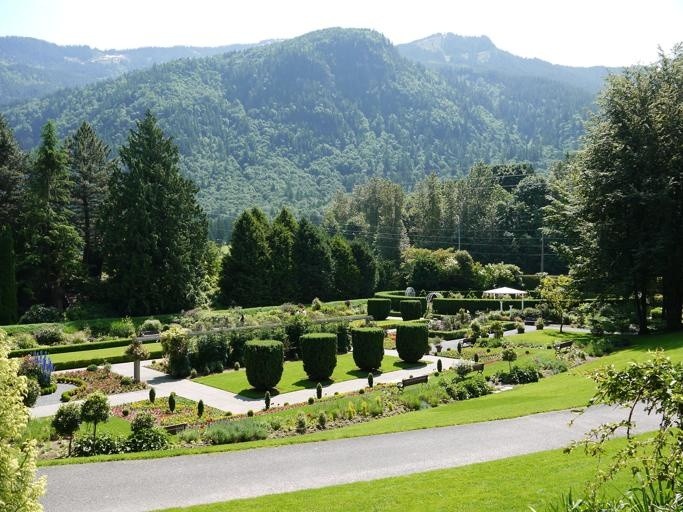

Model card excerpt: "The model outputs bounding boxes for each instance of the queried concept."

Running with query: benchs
[459,364,484,380]
[396,375,428,393]
[163,423,188,436]
[555,340,574,354]
